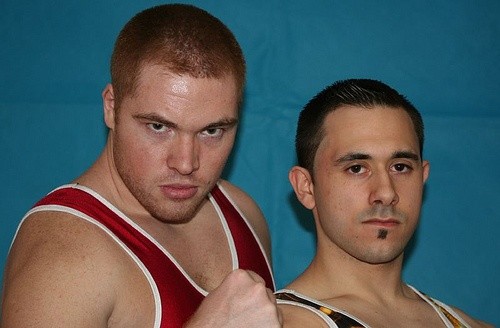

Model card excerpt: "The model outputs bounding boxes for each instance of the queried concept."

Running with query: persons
[1,4,284,328]
[273,79,497,328]
